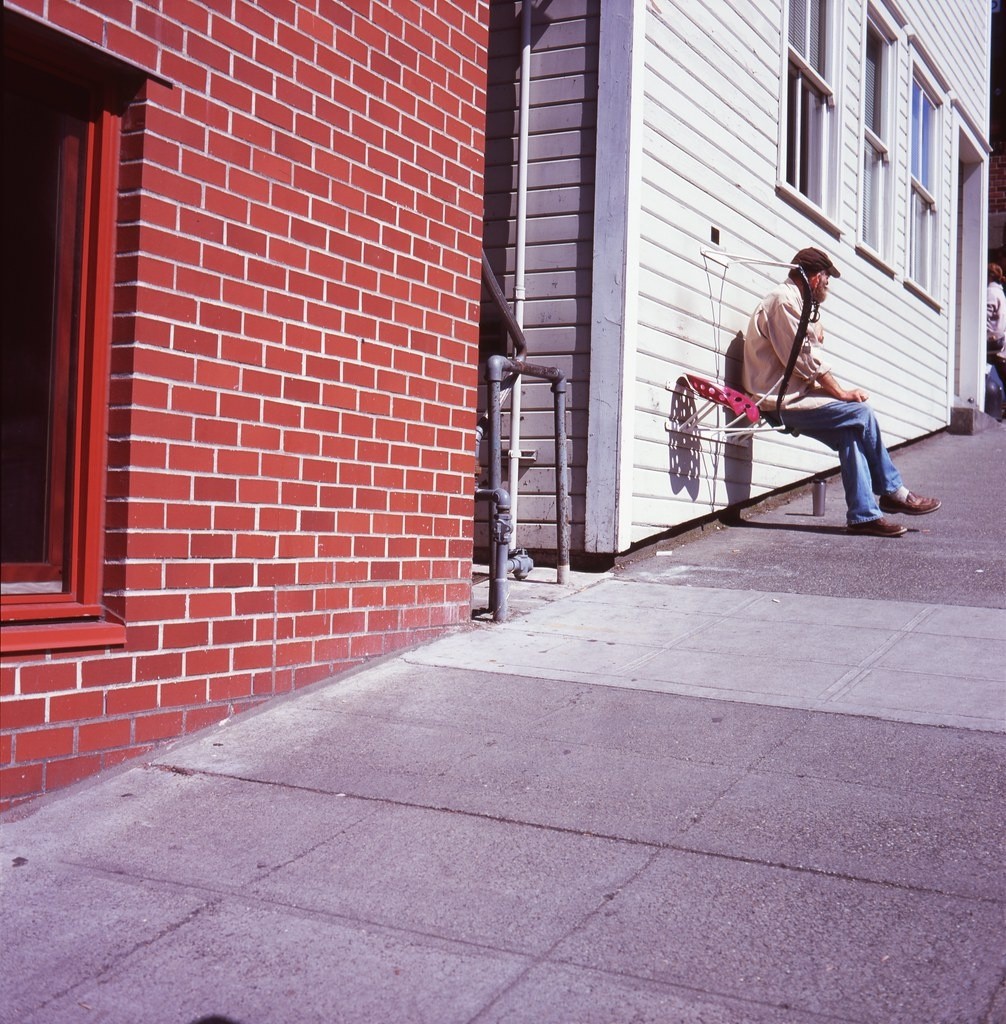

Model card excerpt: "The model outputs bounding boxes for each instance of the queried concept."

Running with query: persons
[987,262,1006,369]
[743,247,943,537]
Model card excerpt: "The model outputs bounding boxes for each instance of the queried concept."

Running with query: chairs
[667,369,793,448]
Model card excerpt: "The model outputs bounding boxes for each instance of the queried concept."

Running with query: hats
[790,247,841,278]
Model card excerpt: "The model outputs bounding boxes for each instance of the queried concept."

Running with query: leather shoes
[878,489,942,515]
[846,517,908,537]
